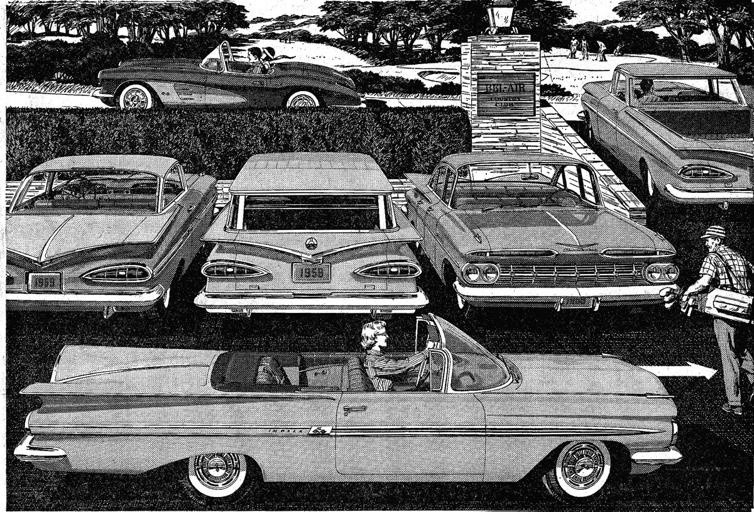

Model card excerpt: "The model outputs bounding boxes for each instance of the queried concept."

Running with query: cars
[577,64,753,211]
[403,152,680,312]
[193,152,430,320]
[6,155,216,321]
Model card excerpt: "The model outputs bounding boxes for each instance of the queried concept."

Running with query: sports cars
[93,40,366,110]
[14,313,683,505]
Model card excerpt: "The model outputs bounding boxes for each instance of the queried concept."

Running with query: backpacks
[690,286,753,324]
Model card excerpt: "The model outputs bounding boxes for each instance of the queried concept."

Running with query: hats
[699,226,727,240]
[261,46,275,60]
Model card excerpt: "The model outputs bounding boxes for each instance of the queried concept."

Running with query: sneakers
[721,390,754,415]
